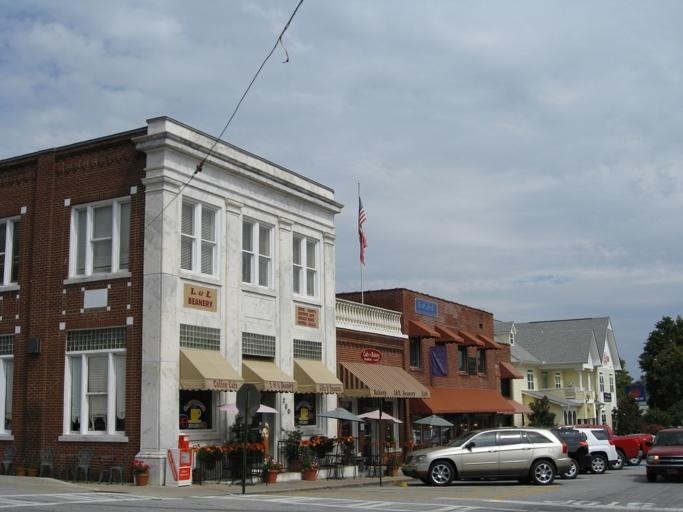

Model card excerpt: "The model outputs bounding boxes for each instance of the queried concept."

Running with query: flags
[357,196,368,267]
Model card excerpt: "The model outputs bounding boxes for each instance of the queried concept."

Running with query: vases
[135,472,149,485]
[16,467,37,477]
[302,470,317,481]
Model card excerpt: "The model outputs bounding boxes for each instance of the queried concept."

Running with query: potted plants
[386,456,400,476]
[263,460,283,484]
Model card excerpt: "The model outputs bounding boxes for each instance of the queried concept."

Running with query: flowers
[280,423,355,470]
[126,459,151,474]
[193,441,266,457]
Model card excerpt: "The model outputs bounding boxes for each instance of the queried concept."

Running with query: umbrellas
[315,406,367,461]
[215,402,280,415]
[412,414,455,427]
[356,409,404,463]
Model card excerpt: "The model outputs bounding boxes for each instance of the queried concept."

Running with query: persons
[258,421,271,459]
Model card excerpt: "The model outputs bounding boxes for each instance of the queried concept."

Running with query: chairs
[38,444,130,485]
[1,445,18,474]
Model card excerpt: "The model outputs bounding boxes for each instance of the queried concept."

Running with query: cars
[401,425,683,486]
